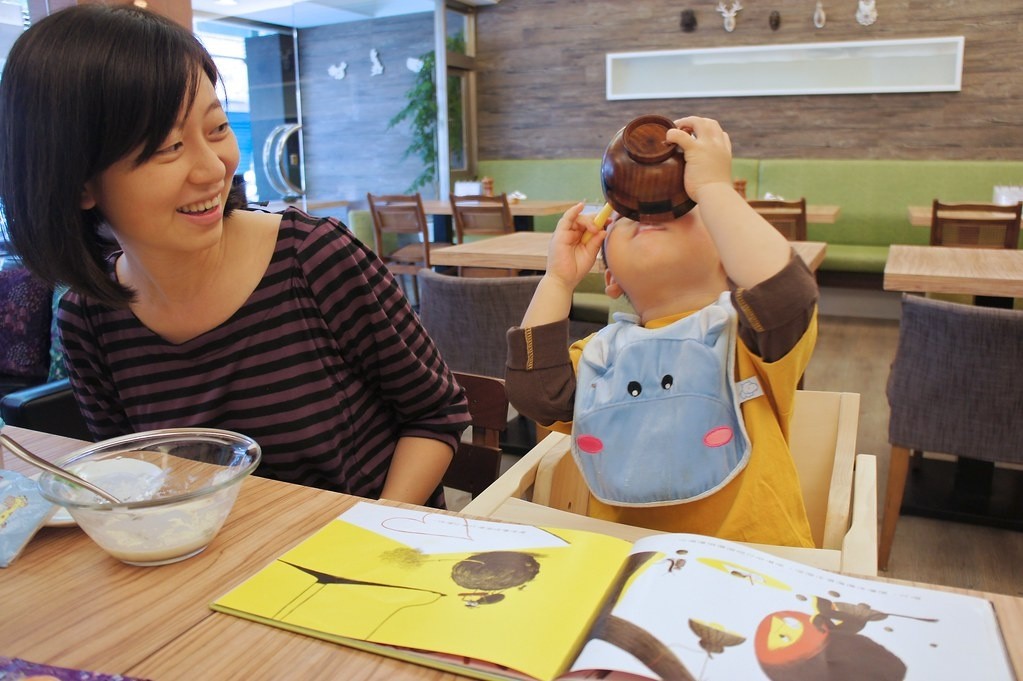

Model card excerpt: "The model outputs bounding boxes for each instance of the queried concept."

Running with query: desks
[429,230,827,453]
[0,424,1023,681]
[752,204,841,225]
[907,205,1023,251]
[388,201,579,242]
[881,243,1023,531]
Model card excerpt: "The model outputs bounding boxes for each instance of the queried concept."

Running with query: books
[212,500,1018,681]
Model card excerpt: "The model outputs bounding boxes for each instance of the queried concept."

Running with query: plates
[27,457,166,527]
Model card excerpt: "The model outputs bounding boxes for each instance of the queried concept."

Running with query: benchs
[453,160,1023,274]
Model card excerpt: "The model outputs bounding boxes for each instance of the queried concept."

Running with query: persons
[503,116,819,549]
[0,4,472,510]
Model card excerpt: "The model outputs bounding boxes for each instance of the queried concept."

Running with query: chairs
[745,198,808,243]
[461,392,878,583]
[877,294,1023,573]
[349,210,419,311]
[418,267,583,450]
[368,190,455,306]
[449,192,524,279]
[929,199,1023,249]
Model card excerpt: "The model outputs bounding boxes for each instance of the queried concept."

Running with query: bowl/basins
[37,428,262,566]
[600,114,697,225]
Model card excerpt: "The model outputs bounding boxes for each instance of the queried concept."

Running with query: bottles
[481,176,494,197]
